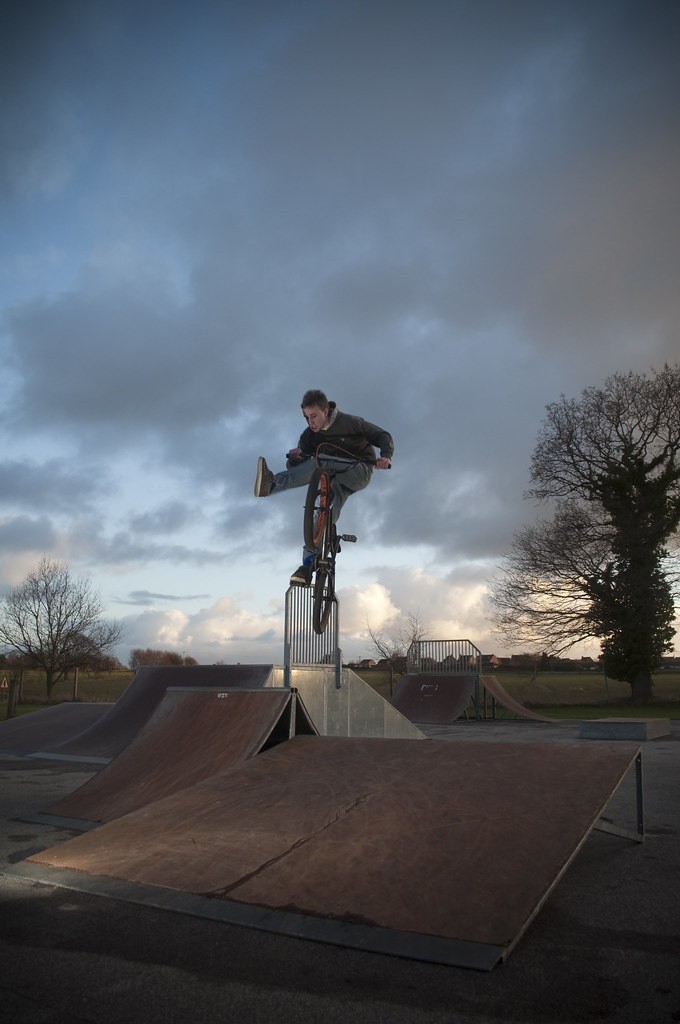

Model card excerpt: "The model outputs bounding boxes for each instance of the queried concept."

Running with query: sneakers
[289,567,309,584]
[254,457,276,497]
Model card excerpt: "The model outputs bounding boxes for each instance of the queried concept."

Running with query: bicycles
[286,441,393,635]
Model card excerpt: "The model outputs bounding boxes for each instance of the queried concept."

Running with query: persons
[253,391,396,584]
